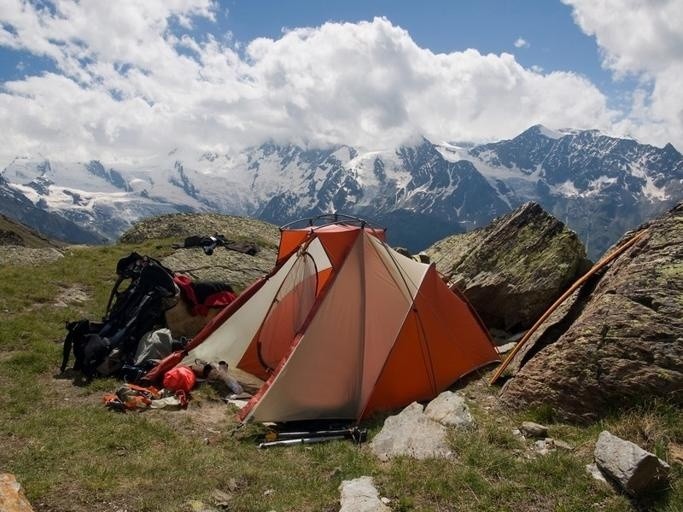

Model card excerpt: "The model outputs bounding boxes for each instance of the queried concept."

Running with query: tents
[140,211,503,430]
